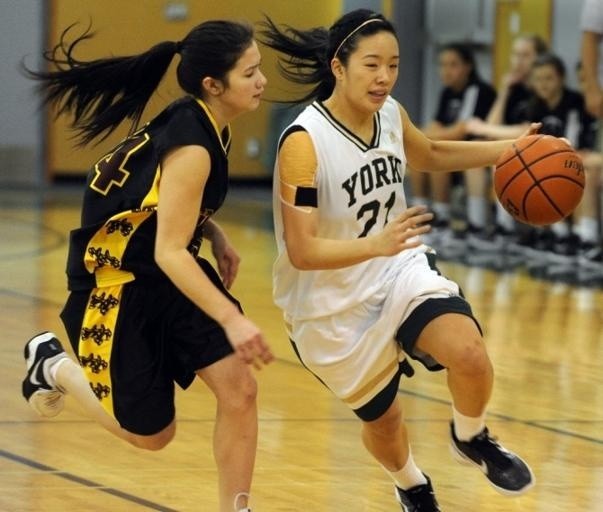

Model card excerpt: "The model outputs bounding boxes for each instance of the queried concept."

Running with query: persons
[271,7,573,512]
[19,17,275,512]
[574,1,603,260]
[509,51,592,254]
[396,38,498,227]
[459,33,549,238]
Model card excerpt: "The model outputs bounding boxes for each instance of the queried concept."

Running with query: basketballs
[495,134,585,225]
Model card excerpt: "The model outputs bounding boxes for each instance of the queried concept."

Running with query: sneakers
[22,330,69,419]
[448,418,536,495]
[419,211,603,270]
[420,232,603,288]
[394,471,441,512]
